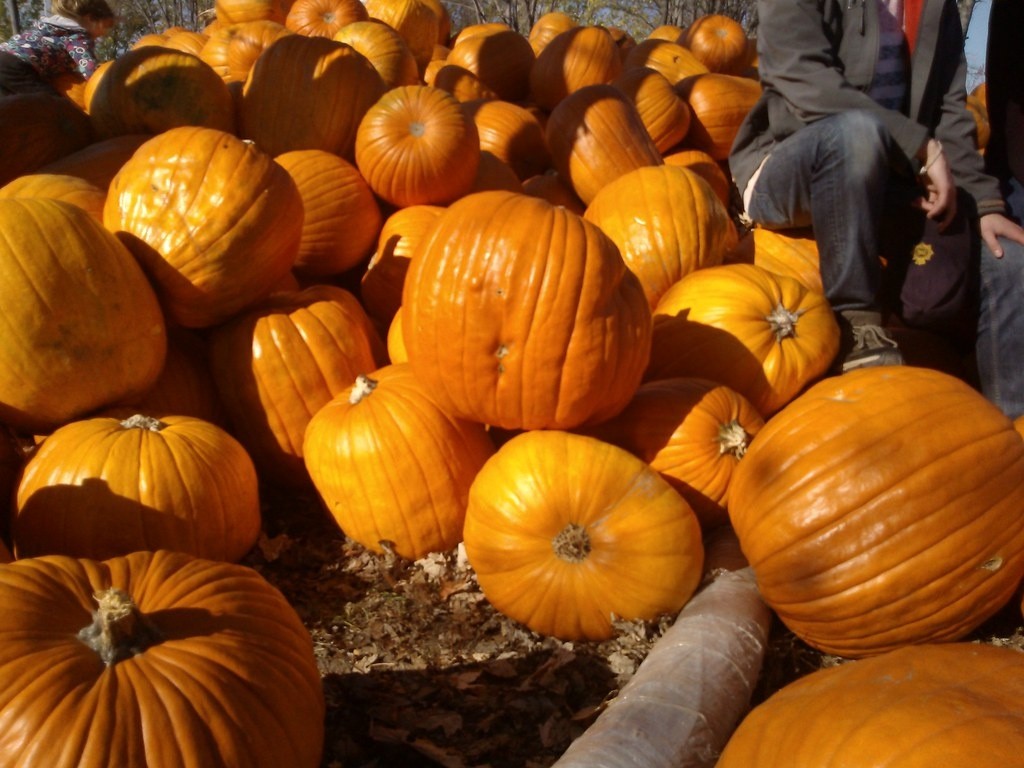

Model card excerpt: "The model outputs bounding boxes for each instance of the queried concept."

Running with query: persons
[0,0,117,98]
[729,0,1024,419]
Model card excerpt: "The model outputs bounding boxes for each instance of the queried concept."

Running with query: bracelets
[918,139,944,176]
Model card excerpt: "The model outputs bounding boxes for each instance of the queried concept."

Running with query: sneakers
[840,310,903,371]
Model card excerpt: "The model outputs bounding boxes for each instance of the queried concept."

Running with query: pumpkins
[0,0,1024,768]
